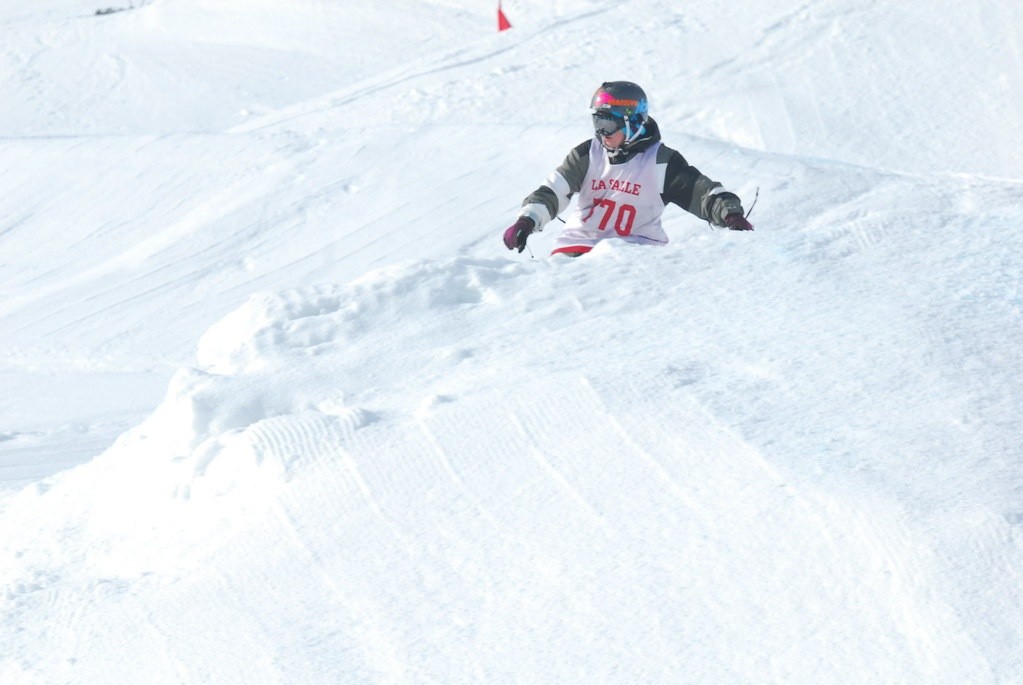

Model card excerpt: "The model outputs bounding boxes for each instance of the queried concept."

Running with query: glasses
[592,113,622,136]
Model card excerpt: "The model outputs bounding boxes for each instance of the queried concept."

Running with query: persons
[503,81,753,255]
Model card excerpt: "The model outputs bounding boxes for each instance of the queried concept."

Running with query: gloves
[503,216,535,253]
[725,213,752,231]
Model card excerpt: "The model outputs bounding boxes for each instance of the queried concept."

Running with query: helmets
[590,81,648,138]
